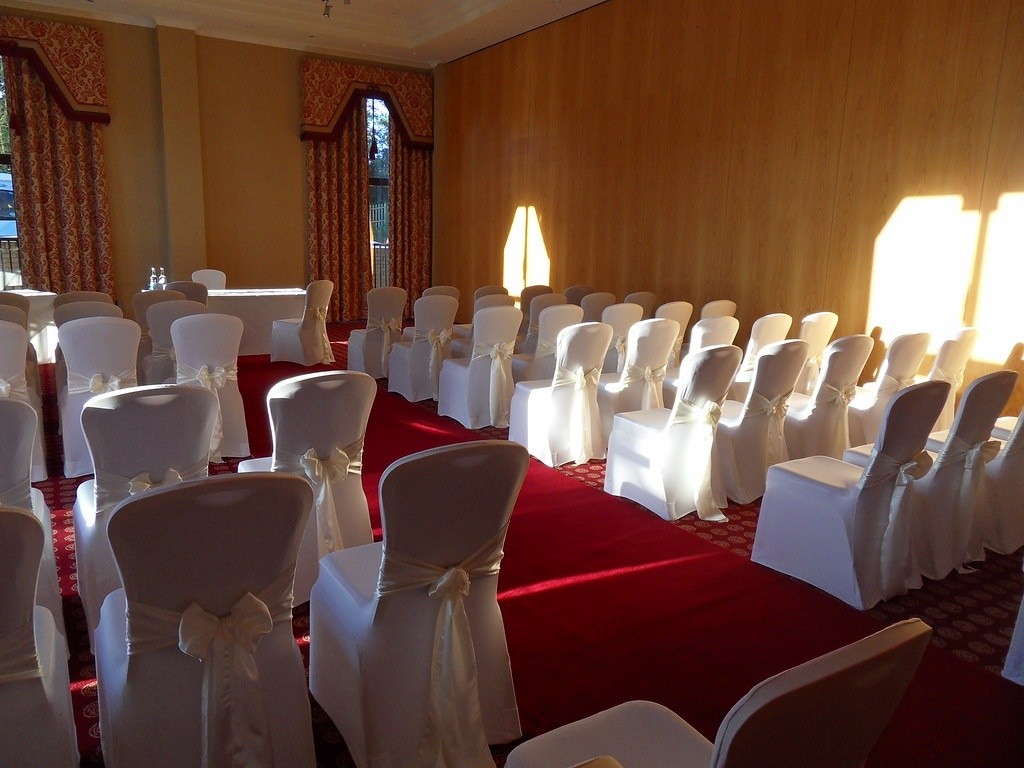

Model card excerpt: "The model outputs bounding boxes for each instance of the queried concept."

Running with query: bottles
[150,268,158,290]
[159,268,168,290]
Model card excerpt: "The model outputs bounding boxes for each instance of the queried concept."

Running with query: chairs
[0,270,1024,611]
[309,440,531,768]
[502,618,934,768]
[1,504,80,768]
[93,471,319,768]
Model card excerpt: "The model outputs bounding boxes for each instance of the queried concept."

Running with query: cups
[154,283,163,291]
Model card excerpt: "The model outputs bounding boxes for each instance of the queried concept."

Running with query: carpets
[213,359,1024,768]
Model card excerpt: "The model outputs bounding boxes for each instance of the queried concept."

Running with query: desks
[1,290,58,339]
[202,288,306,357]
